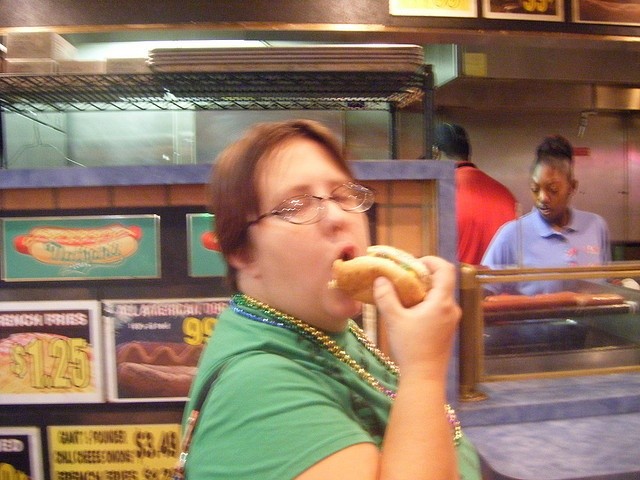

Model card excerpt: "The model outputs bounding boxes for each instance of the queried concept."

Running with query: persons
[479,133,611,340]
[179,117,481,480]
[431,122,517,268]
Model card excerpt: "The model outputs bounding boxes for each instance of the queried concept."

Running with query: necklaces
[229,288,462,448]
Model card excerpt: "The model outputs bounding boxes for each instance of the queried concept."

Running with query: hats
[418,122,469,159]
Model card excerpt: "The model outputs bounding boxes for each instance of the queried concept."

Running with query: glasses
[242,181,376,224]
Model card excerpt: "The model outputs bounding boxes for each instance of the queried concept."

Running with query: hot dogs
[12,224,141,266]
[325,244,431,306]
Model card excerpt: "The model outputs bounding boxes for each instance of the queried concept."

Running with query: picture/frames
[387,0,482,30]
[482,0,568,32]
[570,1,640,36]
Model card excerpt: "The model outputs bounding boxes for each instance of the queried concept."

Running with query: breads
[119,340,205,395]
